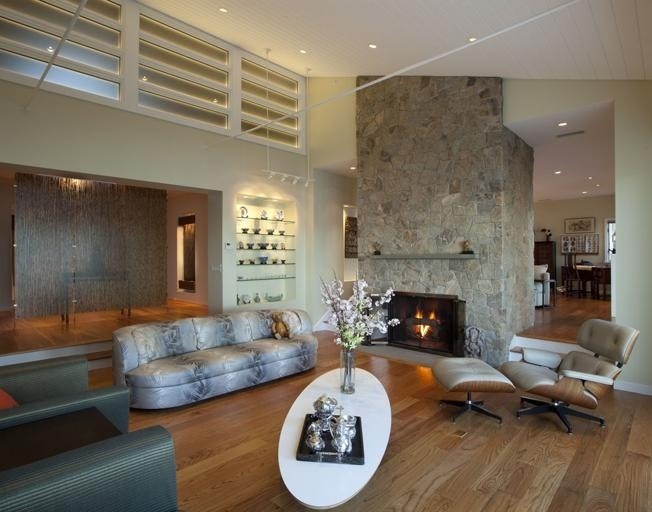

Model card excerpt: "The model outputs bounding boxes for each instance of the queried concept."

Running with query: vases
[339,348,355,394]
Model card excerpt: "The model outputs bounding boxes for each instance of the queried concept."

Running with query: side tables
[0,408,120,472]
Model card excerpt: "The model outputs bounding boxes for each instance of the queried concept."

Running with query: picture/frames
[563,217,596,235]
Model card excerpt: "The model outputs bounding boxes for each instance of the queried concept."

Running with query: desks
[560,265,610,300]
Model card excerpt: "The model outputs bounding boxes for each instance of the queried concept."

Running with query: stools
[431,357,516,424]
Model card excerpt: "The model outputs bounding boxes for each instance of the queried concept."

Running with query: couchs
[111,307,316,410]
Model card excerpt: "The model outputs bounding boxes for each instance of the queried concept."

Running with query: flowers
[314,265,399,391]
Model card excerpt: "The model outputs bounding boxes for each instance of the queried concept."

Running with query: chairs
[499,317,639,434]
[564,253,590,299]
[0,355,128,434]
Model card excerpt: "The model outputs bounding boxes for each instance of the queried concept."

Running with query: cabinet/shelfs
[235,216,296,282]
